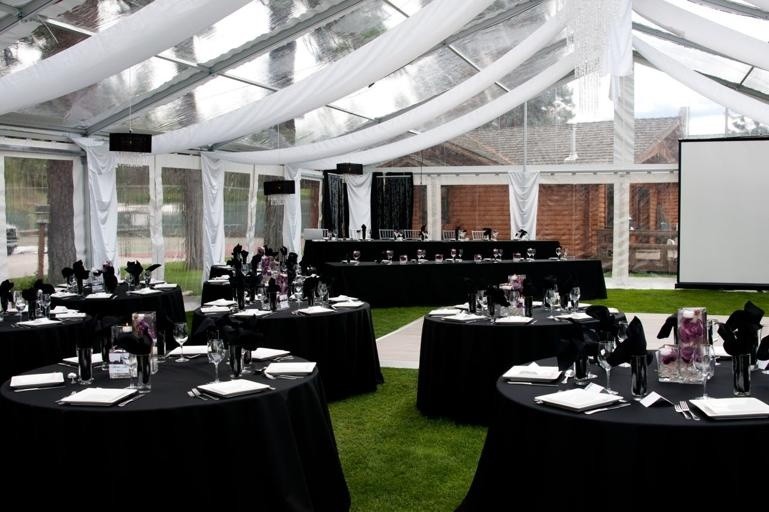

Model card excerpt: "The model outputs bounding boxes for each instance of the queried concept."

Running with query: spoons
[561,368,574,385]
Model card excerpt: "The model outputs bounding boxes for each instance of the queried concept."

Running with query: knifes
[585,403,631,414]
[506,380,559,388]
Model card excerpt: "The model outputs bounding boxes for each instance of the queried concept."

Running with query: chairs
[378,228,489,240]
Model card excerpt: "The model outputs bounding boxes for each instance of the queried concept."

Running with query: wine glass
[9,290,51,320]
[544,287,580,318]
[353,247,568,261]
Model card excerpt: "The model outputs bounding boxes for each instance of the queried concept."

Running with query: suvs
[5,223,18,255]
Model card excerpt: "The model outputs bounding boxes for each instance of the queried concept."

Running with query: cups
[75,321,244,392]
[571,319,753,399]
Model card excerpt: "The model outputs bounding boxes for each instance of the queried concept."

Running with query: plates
[535,389,623,412]
[194,379,271,398]
[8,352,138,405]
[429,300,619,324]
[249,346,319,379]
[689,397,768,419]
[502,364,560,382]
[202,293,364,315]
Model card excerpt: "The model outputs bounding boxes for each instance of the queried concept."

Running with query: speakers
[337,163,363,175]
[263,180,295,195]
[109,132,152,153]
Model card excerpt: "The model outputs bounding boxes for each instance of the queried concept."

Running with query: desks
[304,238,601,304]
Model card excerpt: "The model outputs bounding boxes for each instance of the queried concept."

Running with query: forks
[674,399,699,421]
